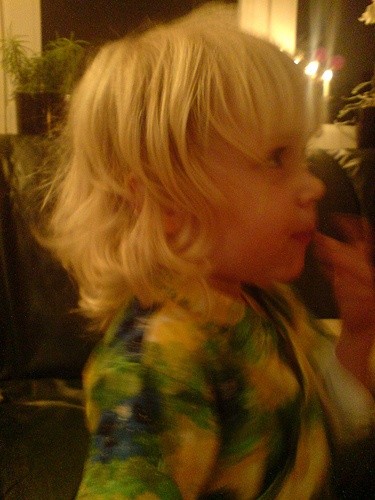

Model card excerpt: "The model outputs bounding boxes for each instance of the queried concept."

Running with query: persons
[26,6,375,500]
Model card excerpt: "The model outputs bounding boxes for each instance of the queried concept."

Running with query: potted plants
[0,22,92,136]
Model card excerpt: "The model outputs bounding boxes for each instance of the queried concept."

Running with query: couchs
[0,132,375,500]
